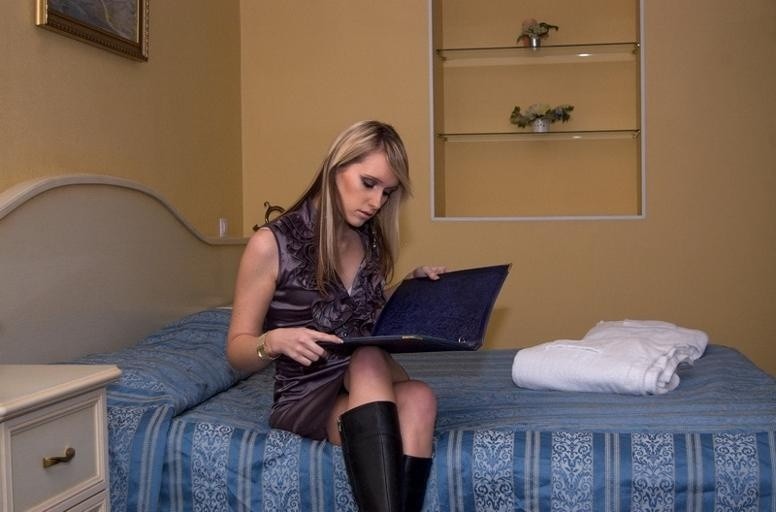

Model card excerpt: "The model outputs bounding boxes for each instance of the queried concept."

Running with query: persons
[225,119,453,512]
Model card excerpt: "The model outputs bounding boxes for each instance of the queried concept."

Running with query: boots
[338,400,434,512]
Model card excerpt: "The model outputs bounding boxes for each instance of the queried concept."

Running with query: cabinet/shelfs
[428,1,645,224]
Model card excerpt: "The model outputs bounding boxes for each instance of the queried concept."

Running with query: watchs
[256,329,281,360]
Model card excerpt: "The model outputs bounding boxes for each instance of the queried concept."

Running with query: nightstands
[1,364,124,512]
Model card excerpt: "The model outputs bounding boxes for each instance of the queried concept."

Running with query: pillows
[68,308,273,414]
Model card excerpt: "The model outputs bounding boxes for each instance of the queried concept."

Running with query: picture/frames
[34,1,149,62]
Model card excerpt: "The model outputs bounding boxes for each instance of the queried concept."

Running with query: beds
[1,176,776,512]
